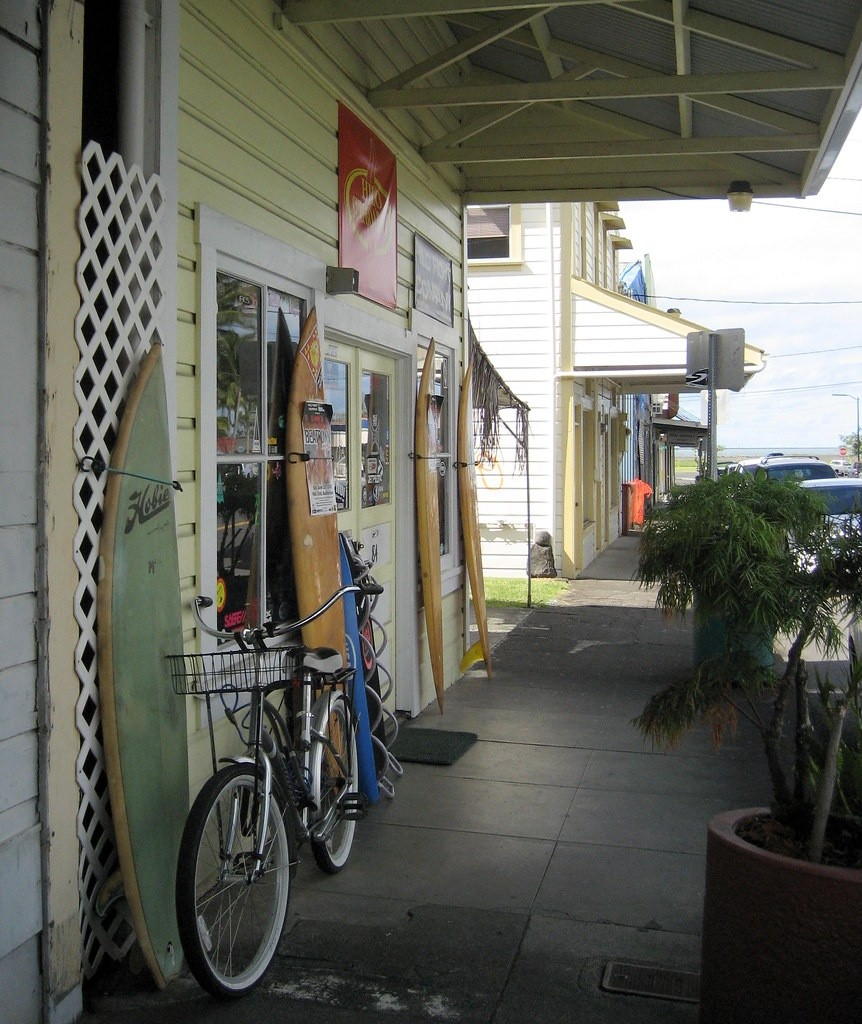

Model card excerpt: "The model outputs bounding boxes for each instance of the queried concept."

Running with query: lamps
[727,180,753,211]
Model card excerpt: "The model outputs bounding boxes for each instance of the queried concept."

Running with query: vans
[831,459,853,474]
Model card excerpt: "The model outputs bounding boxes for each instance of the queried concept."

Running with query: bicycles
[164,580,385,997]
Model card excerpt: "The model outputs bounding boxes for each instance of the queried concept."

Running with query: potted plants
[626,463,862,1024]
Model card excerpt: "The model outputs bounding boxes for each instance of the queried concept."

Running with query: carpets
[387,725,478,768]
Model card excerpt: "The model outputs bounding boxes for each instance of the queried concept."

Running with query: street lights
[832,394,860,461]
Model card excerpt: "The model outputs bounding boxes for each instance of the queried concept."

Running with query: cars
[781,478,862,581]
[695,453,840,481]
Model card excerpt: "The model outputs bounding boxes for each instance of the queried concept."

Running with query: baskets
[164,646,301,695]
